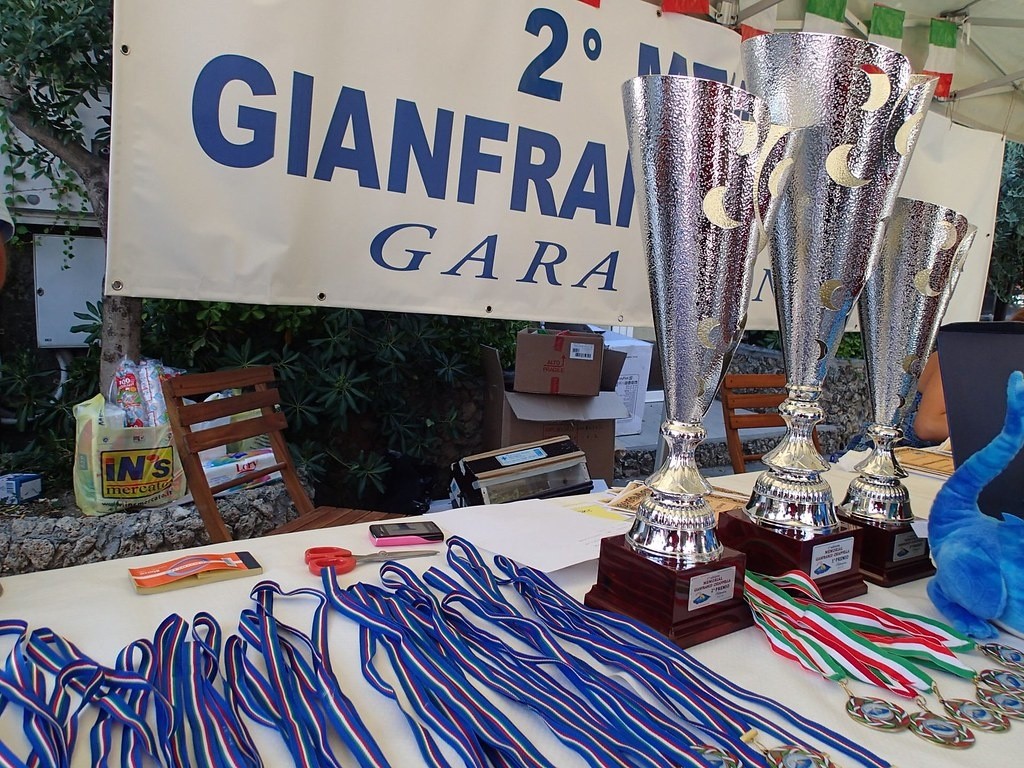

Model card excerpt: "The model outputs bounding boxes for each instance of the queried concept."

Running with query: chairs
[161,366,407,543]
[720,373,821,474]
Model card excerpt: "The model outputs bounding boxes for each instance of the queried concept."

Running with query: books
[128,551,263,597]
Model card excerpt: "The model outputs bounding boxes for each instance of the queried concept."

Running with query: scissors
[305,545,439,576]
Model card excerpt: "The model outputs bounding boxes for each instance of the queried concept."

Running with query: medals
[693,641,1024,768]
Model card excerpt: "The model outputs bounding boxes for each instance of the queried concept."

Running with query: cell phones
[369,521,445,544]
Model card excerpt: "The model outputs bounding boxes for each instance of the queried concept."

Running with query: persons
[828,306,1024,464]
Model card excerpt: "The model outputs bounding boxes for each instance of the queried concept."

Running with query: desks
[0,446,1024,768]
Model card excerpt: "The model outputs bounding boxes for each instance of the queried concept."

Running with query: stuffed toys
[924,367,1024,642]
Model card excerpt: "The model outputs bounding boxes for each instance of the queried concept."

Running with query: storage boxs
[514,327,605,396]
[542,325,654,436]
[459,434,591,504]
[0,473,43,505]
[479,344,632,488]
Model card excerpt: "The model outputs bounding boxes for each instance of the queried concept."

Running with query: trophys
[838,195,980,591]
[584,76,809,649]
[714,29,940,600]
